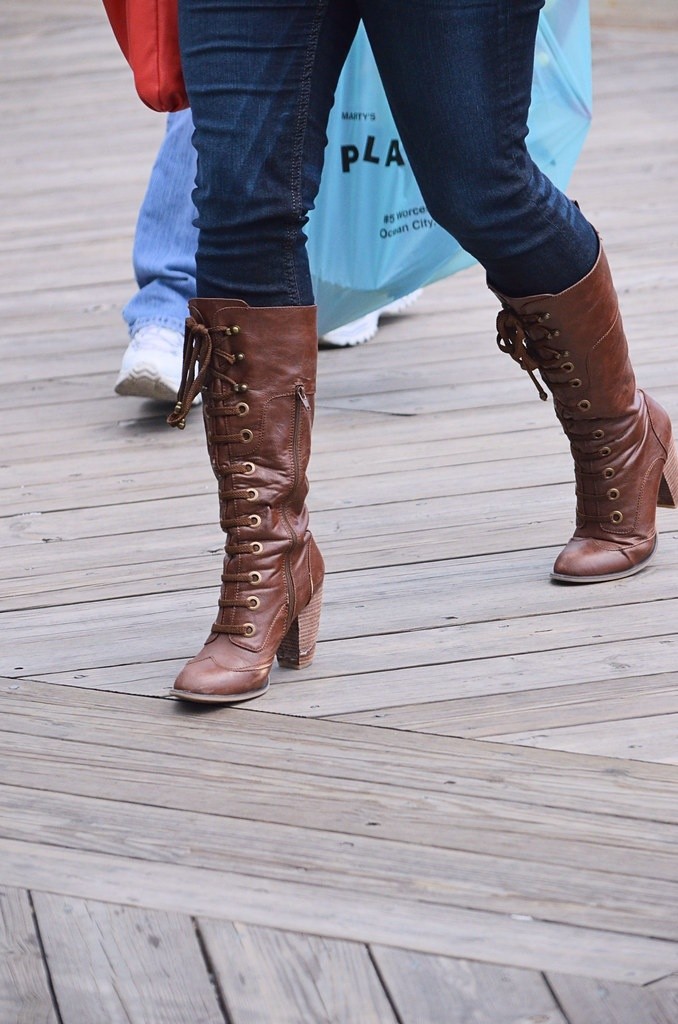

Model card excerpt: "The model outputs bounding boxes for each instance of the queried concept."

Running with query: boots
[487,224,678,584]
[167,300,324,704]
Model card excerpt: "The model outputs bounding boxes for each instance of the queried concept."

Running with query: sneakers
[319,285,423,344]
[115,329,201,407]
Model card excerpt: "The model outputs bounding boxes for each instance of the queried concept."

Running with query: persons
[102,0,677,703]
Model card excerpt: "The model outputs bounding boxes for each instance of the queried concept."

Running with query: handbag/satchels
[101,0,191,113]
